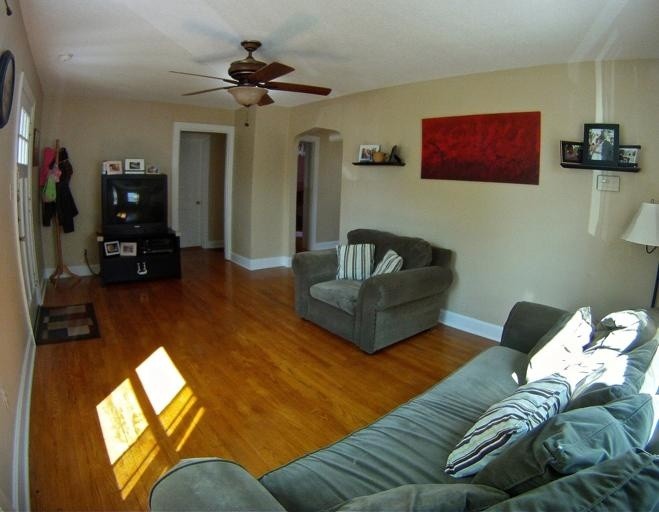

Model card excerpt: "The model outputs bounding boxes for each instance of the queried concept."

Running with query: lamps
[227,41,268,108]
[619,202,659,307]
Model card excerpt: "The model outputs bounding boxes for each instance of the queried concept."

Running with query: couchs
[149,302,659,512]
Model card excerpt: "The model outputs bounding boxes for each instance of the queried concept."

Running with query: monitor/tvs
[101,174,168,236]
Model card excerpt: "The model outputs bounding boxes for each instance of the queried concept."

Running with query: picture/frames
[33,128,41,166]
[582,124,619,167]
[120,242,137,256]
[106,161,122,174]
[561,140,583,165]
[359,145,379,161]
[104,240,120,256]
[618,144,641,167]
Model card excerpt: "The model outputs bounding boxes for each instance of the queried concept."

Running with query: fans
[169,40,331,106]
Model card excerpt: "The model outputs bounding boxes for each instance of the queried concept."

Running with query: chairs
[292,229,453,354]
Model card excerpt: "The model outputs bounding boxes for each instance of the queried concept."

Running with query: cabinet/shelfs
[95,229,181,288]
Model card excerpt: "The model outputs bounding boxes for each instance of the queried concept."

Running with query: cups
[372,151,389,163]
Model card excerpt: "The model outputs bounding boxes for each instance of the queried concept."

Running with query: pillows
[372,249,403,277]
[335,243,376,281]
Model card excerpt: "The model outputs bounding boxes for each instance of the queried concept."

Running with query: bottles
[101,162,107,174]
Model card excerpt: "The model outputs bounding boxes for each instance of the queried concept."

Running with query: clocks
[0,50,16,128]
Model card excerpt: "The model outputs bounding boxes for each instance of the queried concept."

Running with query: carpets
[36,302,101,345]
